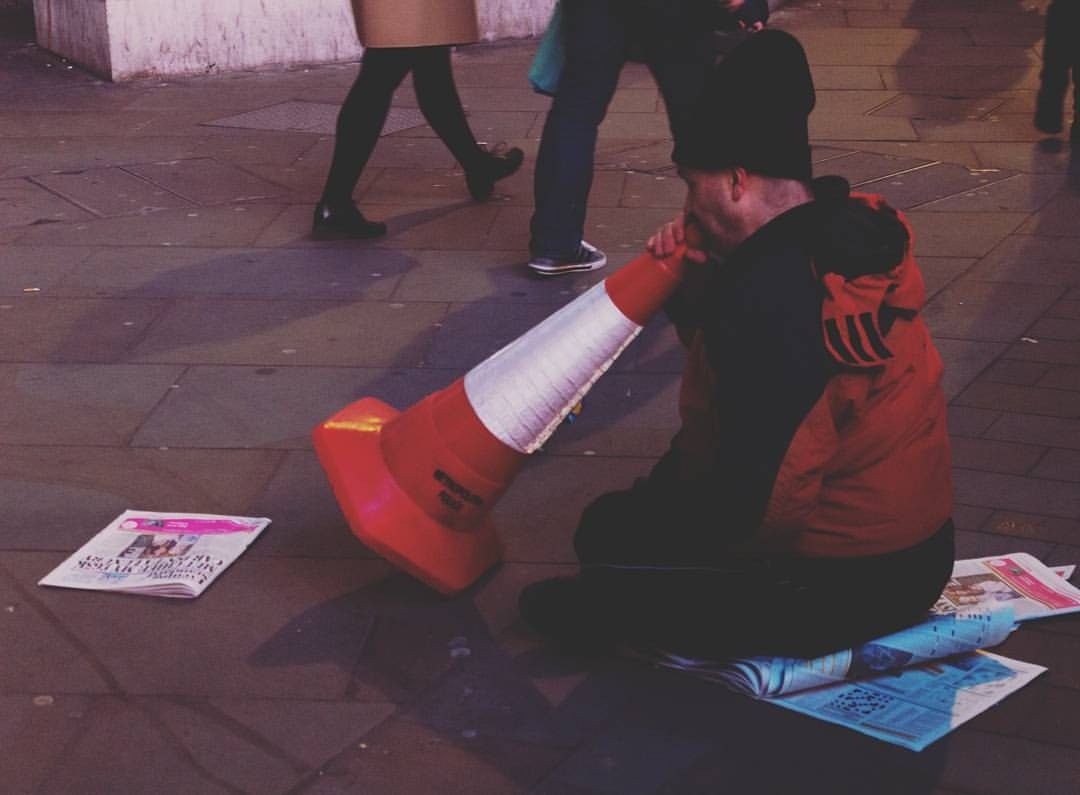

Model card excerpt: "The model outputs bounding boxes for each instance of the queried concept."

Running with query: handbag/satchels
[528,3,565,97]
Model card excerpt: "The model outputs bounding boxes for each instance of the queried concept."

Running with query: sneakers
[312,199,387,240]
[467,141,524,200]
[527,240,608,276]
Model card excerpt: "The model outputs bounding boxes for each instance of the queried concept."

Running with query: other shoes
[1033,95,1066,134]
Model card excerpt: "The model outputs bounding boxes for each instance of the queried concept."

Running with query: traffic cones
[305,218,707,595]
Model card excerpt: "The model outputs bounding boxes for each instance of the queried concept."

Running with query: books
[38,511,272,598]
[657,605,1048,753]
[928,552,1080,621]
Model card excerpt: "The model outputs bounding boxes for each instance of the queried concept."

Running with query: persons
[314,0,523,240]
[944,575,1020,603]
[1035,0,1080,133]
[516,29,955,658]
[529,1,770,274]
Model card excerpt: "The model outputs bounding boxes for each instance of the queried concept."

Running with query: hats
[672,23,817,181]
